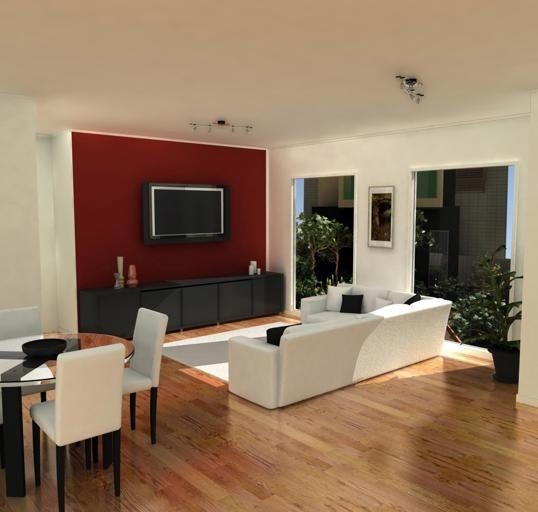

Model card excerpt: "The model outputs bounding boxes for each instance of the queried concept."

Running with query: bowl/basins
[22,352,65,368]
[23,337,67,360]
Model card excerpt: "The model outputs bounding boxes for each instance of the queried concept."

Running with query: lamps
[395,75,424,104]
[186,122,253,135]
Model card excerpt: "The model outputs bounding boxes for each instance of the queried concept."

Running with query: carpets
[162,322,299,384]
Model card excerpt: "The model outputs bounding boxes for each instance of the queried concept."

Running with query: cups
[250,261,257,273]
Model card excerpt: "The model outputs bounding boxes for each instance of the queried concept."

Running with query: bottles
[256,268,260,275]
[249,264,255,275]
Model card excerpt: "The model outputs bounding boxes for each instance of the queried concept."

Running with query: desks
[0,332,135,496]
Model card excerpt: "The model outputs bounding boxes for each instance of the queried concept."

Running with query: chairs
[0,305,46,470]
[29,343,125,512]
[92,307,170,464]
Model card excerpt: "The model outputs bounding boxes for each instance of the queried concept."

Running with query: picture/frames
[367,185,395,249]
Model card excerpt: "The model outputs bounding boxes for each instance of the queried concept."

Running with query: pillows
[325,283,352,311]
[404,294,422,304]
[266,321,302,346]
[375,297,392,309]
[340,294,363,313]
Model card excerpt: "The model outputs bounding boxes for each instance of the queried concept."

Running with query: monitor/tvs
[149,183,224,240]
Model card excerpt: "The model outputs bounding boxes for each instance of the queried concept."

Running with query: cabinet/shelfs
[78,271,283,341]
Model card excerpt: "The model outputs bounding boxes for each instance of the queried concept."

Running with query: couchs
[229,292,453,409]
[301,282,390,323]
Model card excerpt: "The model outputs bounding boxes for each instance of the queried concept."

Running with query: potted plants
[450,243,525,382]
